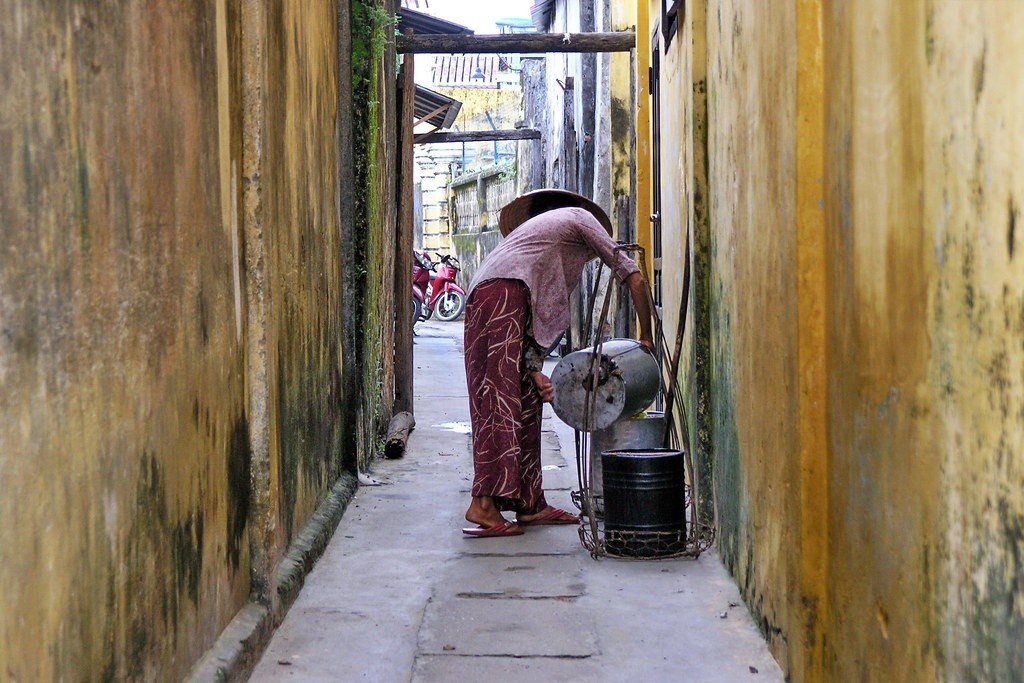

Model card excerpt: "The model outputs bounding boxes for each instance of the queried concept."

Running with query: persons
[464,189,653,536]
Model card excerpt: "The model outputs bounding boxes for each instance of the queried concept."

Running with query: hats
[499,189,613,262]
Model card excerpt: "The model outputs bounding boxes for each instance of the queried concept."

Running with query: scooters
[420,251,467,322]
[410,247,440,337]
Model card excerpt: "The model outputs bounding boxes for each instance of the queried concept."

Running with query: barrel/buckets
[548,337,689,558]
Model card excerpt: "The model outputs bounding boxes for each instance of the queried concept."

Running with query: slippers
[511,508,580,525]
[462,521,525,535]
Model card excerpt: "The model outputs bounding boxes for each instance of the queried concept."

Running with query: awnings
[400,9,475,35]
[414,84,463,143]
